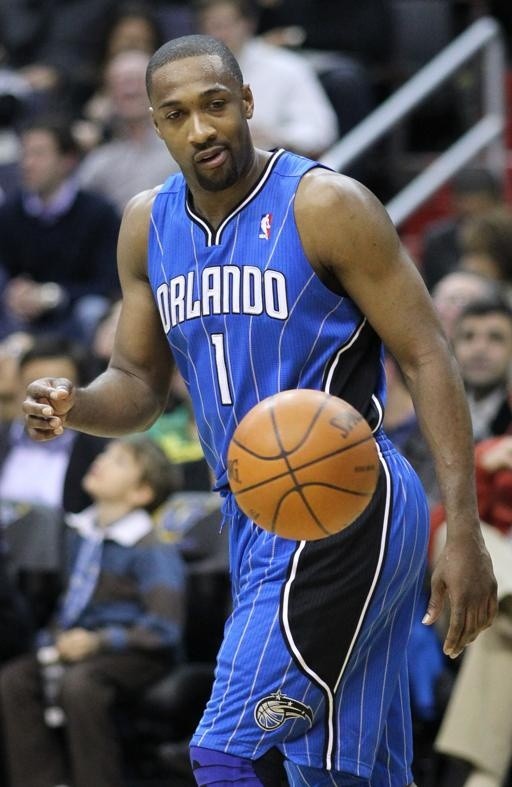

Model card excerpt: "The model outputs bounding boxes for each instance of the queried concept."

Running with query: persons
[0,0,512,787]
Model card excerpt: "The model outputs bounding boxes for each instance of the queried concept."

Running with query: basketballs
[226,388,380,540]
